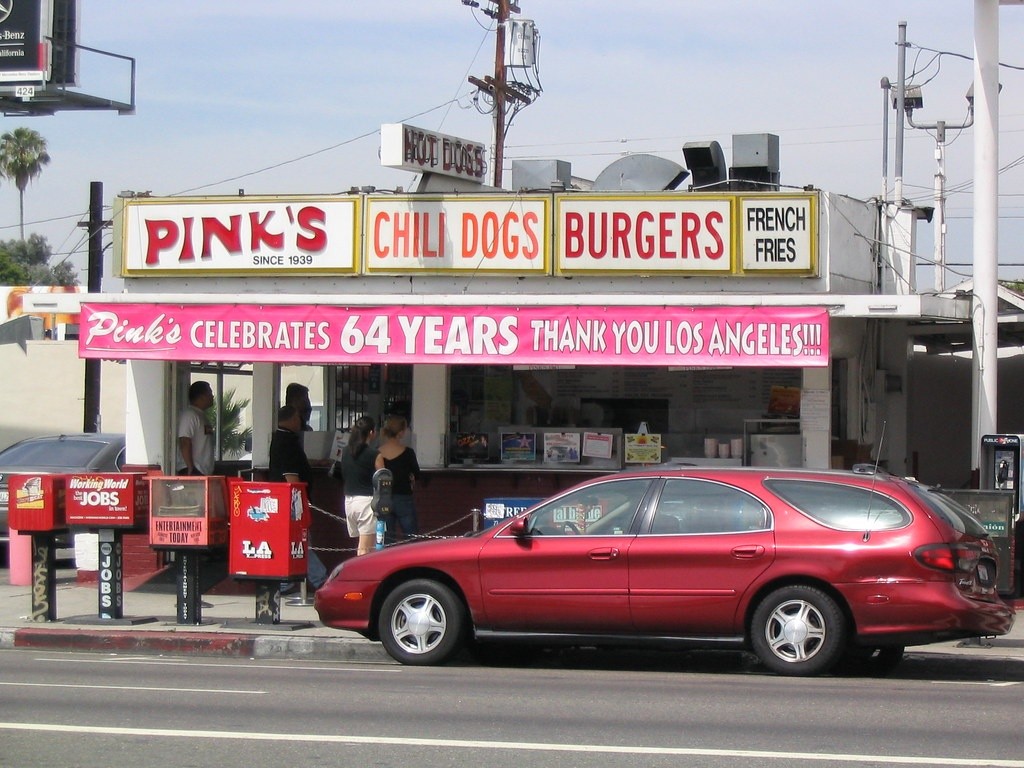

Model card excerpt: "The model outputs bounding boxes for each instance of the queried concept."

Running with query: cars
[315,467,1014,676]
[0,432,129,570]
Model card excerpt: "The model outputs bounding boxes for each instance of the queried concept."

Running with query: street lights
[888,81,1004,293]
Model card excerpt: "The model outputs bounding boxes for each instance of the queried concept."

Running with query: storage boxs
[832,456,844,469]
[769,384,800,417]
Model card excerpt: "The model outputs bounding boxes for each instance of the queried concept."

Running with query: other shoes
[201,601,214,608]
[282,586,298,595]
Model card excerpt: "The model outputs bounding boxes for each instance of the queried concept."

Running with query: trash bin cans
[943,490,1015,594]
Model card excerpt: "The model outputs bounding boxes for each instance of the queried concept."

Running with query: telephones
[994,447,1016,490]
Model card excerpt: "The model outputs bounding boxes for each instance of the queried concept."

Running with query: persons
[284,383,313,430]
[376,413,424,548]
[269,405,327,592]
[177,381,217,562]
[340,415,386,557]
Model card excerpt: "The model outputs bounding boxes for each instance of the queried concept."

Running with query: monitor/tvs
[453,432,489,465]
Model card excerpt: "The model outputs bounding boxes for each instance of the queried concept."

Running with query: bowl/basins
[704,438,743,458]
